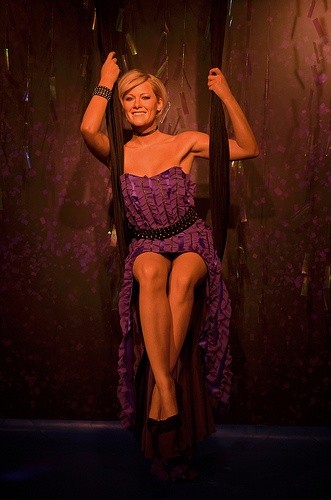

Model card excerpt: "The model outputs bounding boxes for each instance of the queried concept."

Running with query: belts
[133,208,200,240]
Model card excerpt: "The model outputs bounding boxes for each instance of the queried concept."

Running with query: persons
[80,51,260,435]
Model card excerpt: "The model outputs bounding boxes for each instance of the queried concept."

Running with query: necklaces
[131,125,158,136]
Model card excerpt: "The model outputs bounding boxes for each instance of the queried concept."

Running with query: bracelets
[91,86,111,101]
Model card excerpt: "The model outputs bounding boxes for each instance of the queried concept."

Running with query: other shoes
[142,381,183,460]
